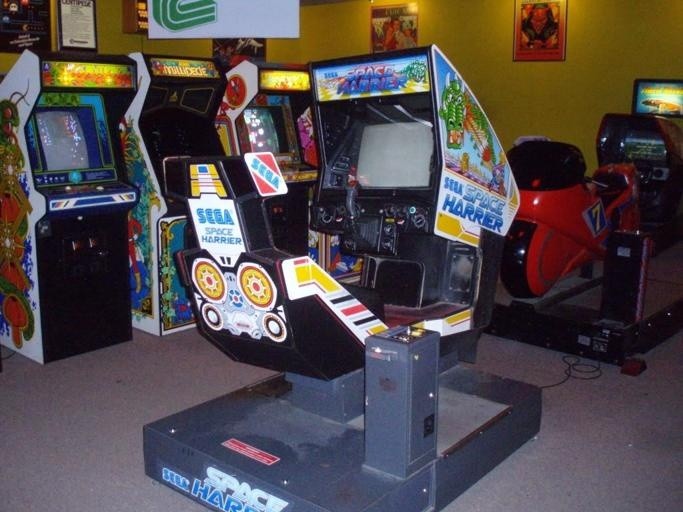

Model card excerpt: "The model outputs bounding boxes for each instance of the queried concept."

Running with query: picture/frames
[370,3,418,54]
[513,0,568,61]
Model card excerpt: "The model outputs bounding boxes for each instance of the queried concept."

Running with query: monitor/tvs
[35,109,90,171]
[242,105,282,152]
[350,114,433,189]
[618,132,667,160]
